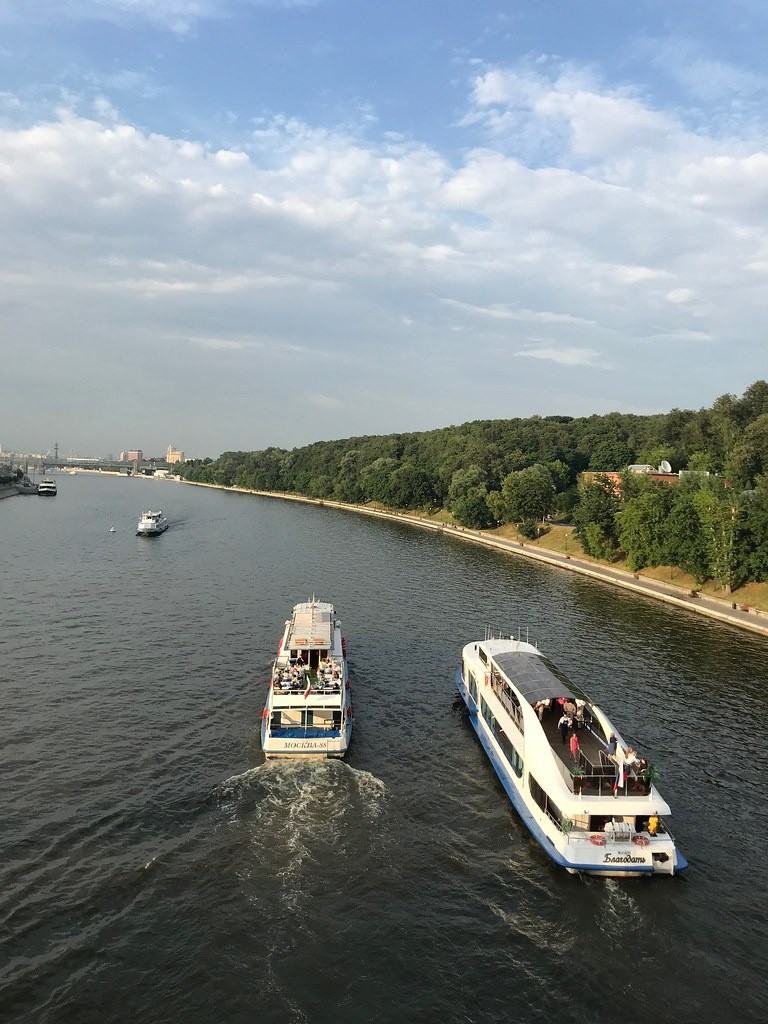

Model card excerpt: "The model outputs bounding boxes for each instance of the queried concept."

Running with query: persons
[275,655,341,694]
[535,697,647,790]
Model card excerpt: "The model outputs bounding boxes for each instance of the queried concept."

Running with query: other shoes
[575,760,577,764]
[569,756,572,760]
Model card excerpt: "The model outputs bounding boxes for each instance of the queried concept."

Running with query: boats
[453,622,689,877]
[133,508,170,537]
[14,476,58,497]
[261,591,353,763]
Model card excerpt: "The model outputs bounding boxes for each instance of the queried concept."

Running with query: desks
[574,715,588,729]
[273,663,341,693]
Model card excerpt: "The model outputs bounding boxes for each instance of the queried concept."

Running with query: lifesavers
[633,835,650,845]
[590,834,606,845]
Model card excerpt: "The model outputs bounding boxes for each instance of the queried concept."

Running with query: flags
[303,676,311,700]
[612,761,627,793]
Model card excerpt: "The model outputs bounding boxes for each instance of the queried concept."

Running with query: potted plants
[569,765,587,790]
